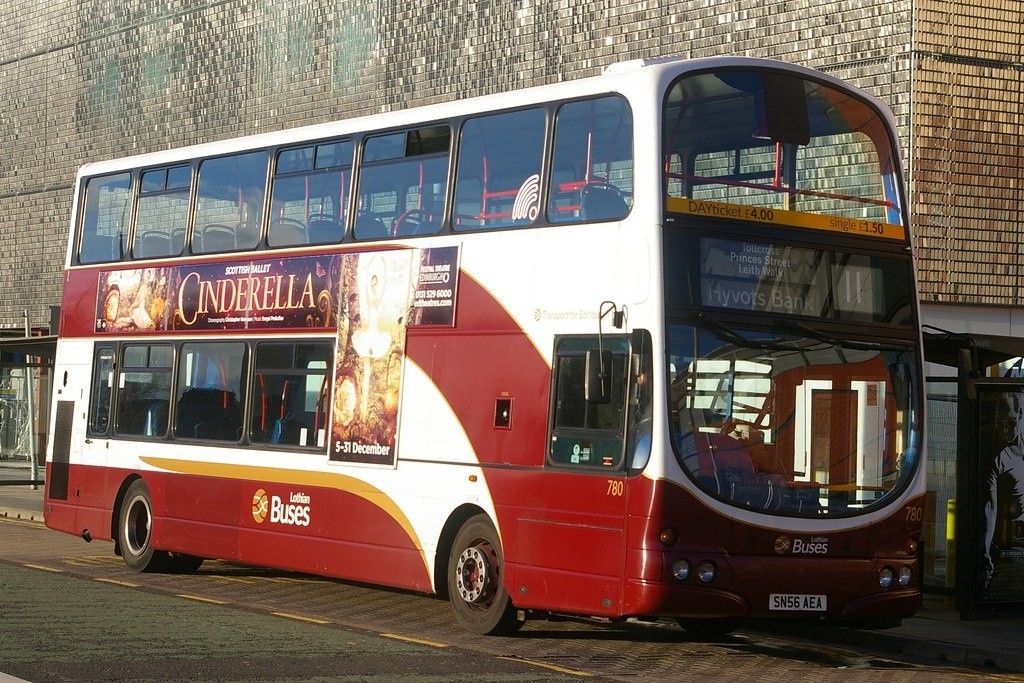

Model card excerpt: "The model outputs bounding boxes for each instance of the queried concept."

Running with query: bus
[40,57,982,640]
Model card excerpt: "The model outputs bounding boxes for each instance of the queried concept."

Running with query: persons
[572,360,654,458]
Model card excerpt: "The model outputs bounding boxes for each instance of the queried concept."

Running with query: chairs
[142,398,312,447]
[109,210,456,263]
